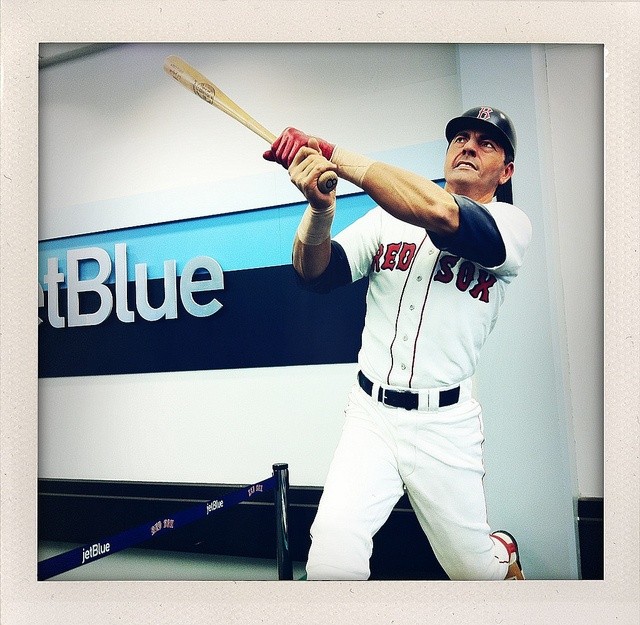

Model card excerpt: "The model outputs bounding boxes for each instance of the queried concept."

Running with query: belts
[356,369,460,410]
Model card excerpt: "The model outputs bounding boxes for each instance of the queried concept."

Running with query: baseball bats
[163,54,338,194]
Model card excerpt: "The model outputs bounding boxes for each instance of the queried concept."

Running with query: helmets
[445,106,517,162]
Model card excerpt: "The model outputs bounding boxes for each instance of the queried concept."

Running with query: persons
[263,106,531,579]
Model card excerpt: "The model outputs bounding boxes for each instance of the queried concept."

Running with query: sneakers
[491,530,526,580]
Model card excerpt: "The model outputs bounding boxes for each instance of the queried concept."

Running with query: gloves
[262,126,335,169]
